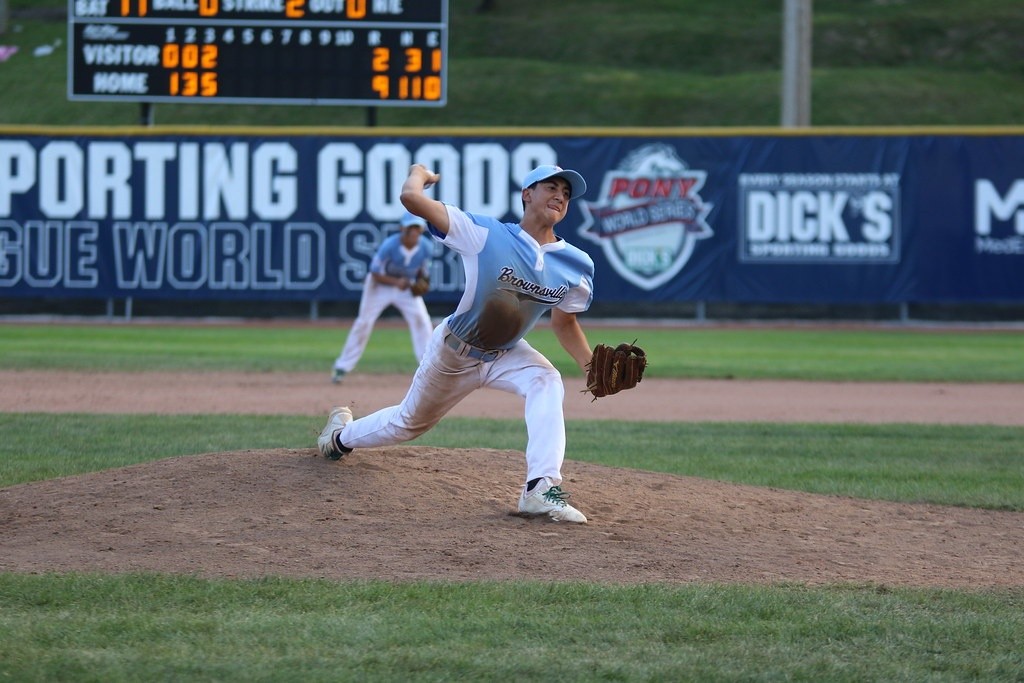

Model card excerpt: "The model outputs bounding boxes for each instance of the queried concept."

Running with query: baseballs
[423,170,436,189]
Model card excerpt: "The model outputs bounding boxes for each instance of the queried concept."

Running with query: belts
[445,335,497,363]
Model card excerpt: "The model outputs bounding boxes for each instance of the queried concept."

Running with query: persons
[317,163,647,524]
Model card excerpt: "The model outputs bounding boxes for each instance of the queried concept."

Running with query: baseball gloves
[586,342,647,398]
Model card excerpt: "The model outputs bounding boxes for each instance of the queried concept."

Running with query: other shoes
[329,365,345,385]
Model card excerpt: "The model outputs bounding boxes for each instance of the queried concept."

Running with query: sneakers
[517,477,588,524]
[317,407,353,460]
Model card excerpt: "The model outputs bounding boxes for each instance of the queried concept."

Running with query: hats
[523,164,587,200]
[400,211,428,231]
